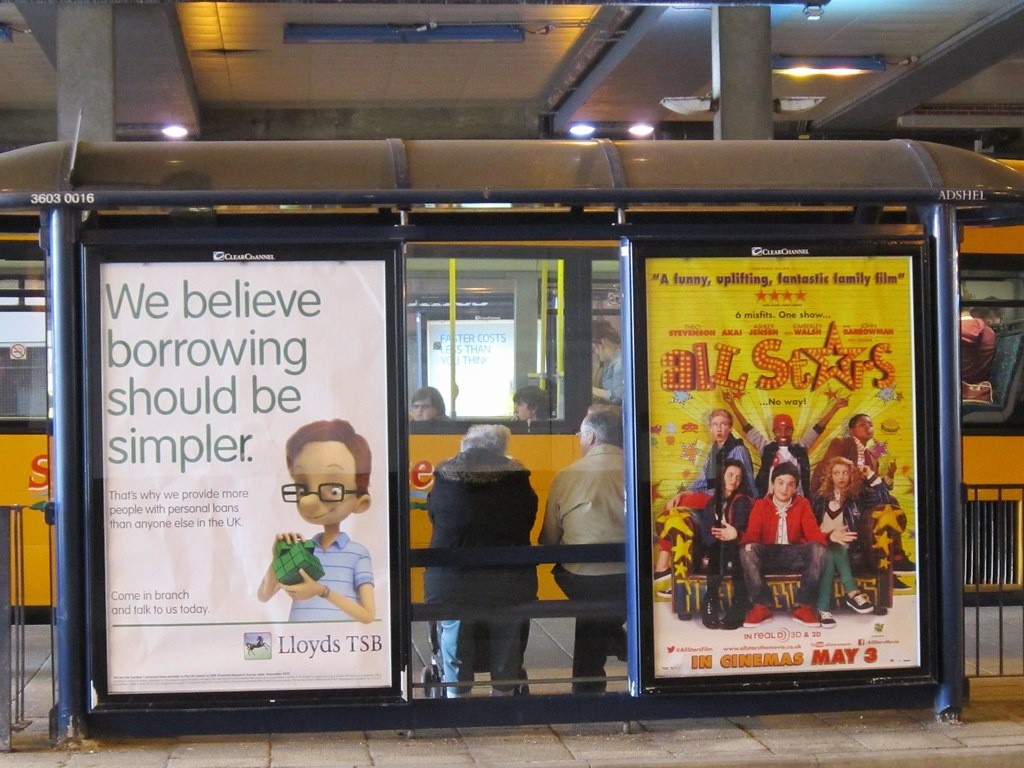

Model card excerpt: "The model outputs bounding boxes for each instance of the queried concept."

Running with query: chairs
[960,320,1024,428]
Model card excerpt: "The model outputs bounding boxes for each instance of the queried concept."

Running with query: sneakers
[818,609,836,628]
[846,593,874,614]
[893,557,916,575]
[654,567,672,584]
[657,587,672,599]
[743,604,773,627]
[792,606,820,627]
[893,574,913,591]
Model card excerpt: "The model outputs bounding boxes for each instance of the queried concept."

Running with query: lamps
[282,23,525,45]
[772,95,827,114]
[772,56,887,73]
[660,96,719,115]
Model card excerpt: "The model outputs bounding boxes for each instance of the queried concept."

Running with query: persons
[590,318,624,405]
[512,385,550,422]
[651,389,916,630]
[424,424,538,700]
[410,386,447,423]
[960,287,1006,384]
[539,404,629,691]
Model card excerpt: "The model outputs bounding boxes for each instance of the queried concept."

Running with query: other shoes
[617,642,628,662]
[489,689,514,697]
[446,692,473,698]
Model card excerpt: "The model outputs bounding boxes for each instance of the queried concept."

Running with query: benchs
[408,540,627,622]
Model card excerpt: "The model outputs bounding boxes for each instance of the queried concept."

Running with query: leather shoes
[699,601,720,629]
[719,607,746,629]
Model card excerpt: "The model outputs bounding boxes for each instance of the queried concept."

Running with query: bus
[0,138,1024,619]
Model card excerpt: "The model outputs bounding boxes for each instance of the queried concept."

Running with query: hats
[772,414,794,431]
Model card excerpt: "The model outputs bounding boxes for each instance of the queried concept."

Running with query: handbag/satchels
[962,380,993,404]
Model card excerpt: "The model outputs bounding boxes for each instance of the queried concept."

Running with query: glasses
[412,402,431,409]
[576,430,588,437]
